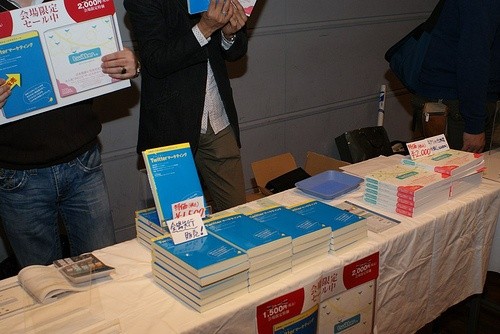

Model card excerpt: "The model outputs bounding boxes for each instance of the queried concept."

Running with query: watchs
[132,59,142,79]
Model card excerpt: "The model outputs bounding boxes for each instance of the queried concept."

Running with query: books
[187,0,258,17]
[134,141,488,314]
[0,0,133,126]
[0,253,116,316]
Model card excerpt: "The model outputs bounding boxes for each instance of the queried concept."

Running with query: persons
[421,0,500,154]
[0,0,139,270]
[123,0,248,213]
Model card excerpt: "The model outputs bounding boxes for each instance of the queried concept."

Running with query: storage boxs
[251,151,352,196]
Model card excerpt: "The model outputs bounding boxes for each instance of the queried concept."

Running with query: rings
[121,67,126,75]
[222,11,228,15]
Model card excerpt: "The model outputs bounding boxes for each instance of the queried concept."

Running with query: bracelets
[226,34,237,42]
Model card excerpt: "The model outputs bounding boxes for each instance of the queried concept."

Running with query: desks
[0,134,500,334]
